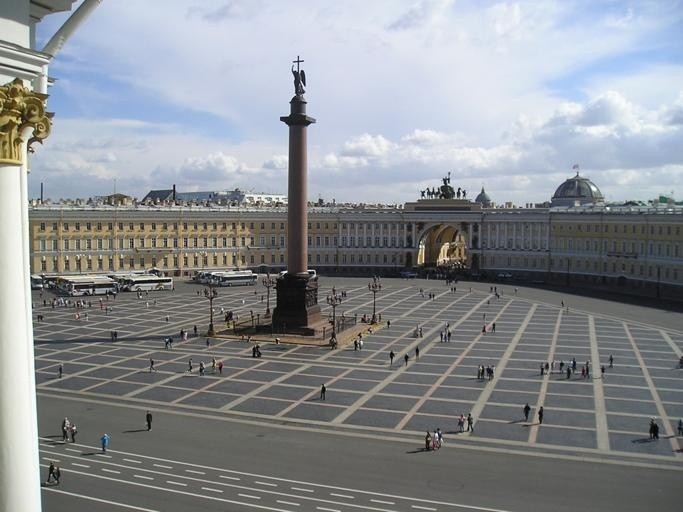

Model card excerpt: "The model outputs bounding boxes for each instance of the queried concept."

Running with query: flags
[571,163,579,170]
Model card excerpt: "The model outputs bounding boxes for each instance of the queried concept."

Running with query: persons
[524,403,531,420]
[675,418,682,435]
[101,434,110,452]
[110,316,260,376]
[290,62,305,96]
[146,410,153,431]
[225,311,233,323]
[677,355,682,369]
[320,383,325,400]
[458,413,473,433]
[165,314,169,321]
[325,259,500,366]
[32,279,158,321]
[561,300,564,307]
[647,416,655,441]
[477,364,494,381]
[652,423,659,440]
[43,461,54,486]
[62,417,77,443]
[540,354,613,379]
[58,364,63,378]
[419,175,467,200]
[425,428,442,450]
[53,466,61,486]
[538,406,543,424]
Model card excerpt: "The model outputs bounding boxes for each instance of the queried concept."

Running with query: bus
[191,270,257,287]
[31,272,174,297]
[279,269,317,279]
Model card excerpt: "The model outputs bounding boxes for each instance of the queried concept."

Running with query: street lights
[203,279,217,335]
[368,275,383,322]
[262,272,274,319]
[327,286,347,341]
